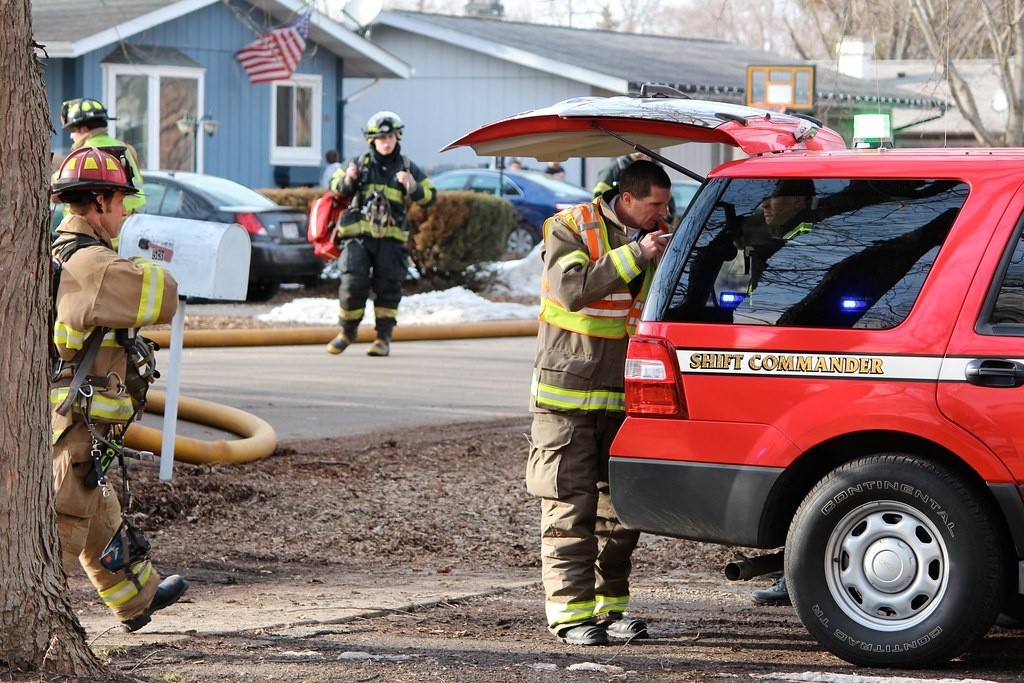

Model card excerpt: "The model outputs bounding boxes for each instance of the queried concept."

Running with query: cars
[429,169,597,261]
[133,170,324,303]
[668,180,702,222]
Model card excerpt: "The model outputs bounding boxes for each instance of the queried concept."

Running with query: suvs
[439,79,1024,666]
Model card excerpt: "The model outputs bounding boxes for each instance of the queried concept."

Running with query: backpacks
[307,190,351,260]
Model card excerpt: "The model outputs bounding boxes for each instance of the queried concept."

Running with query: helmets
[50,147,139,192]
[363,111,405,140]
[762,179,816,196]
[59,98,119,130]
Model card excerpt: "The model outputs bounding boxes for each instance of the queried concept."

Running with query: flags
[233,10,312,85]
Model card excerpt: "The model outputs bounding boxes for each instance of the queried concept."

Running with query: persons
[509,151,817,308]
[526,161,677,643]
[326,111,437,356]
[48,149,190,633]
[50,97,146,253]
[320,148,343,189]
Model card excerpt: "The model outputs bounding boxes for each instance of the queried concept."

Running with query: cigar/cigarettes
[659,233,673,238]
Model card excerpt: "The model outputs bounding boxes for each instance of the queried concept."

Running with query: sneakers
[757,569,783,580]
[751,576,792,606]
[327,328,357,354]
[598,619,648,639]
[121,574,189,633]
[367,338,390,356]
[559,621,609,645]
